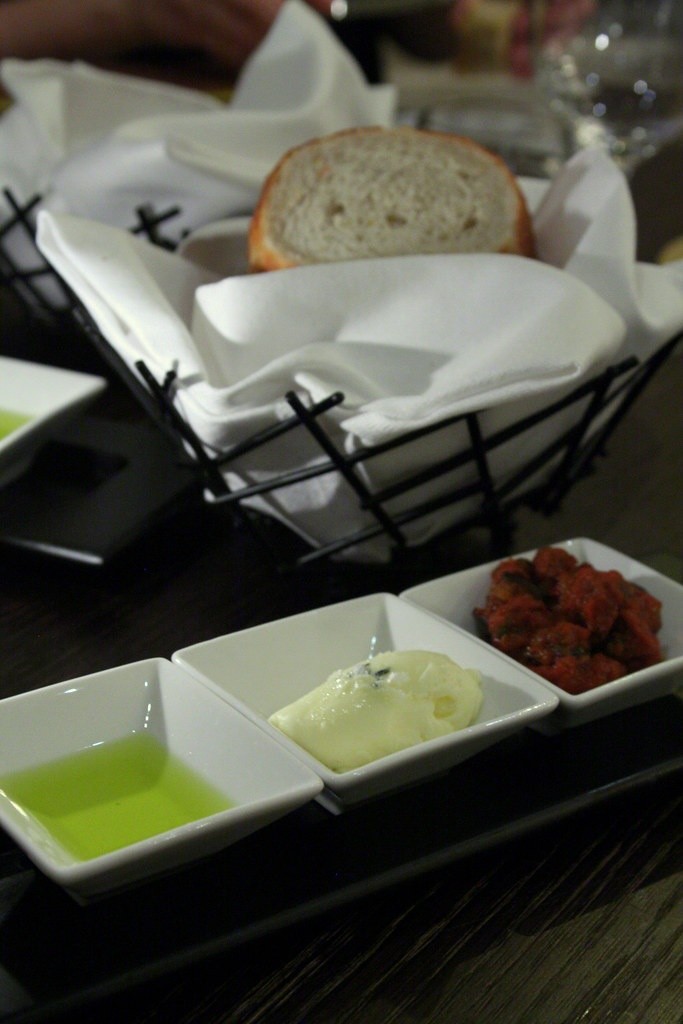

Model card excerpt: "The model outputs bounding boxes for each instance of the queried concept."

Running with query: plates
[0,657,324,898]
[398,536,683,727]
[0,356,106,483]
[170,593,560,809]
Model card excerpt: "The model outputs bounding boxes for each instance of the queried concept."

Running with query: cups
[533,0,683,179]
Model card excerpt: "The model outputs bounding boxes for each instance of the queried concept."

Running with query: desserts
[268,649,486,774]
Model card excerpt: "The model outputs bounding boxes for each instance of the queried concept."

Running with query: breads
[247,124,538,272]
